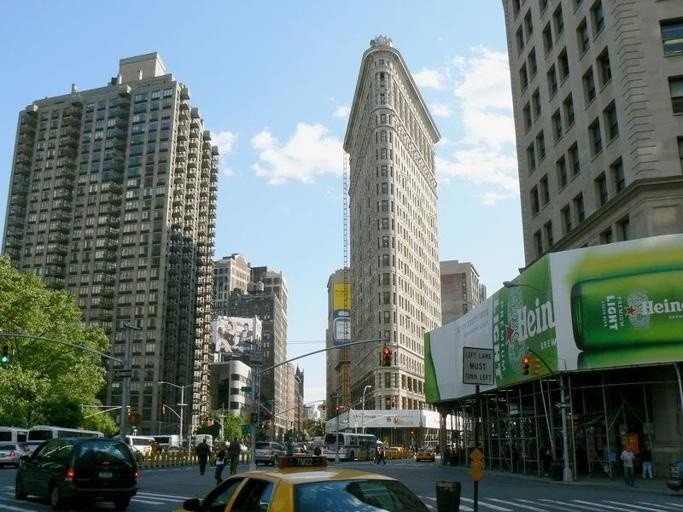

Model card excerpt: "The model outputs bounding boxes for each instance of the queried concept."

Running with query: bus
[1,424,211,468]
[323,432,376,461]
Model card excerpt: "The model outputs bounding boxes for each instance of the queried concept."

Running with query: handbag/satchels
[215,457,225,465]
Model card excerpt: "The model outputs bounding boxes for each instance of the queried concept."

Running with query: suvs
[13,436,138,511]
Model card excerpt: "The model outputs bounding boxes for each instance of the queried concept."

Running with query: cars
[174,454,437,511]
[212,436,323,468]
[667,459,682,494]
[387,439,463,461]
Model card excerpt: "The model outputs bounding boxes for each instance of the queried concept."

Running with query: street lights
[362,384,372,434]
[156,380,204,451]
[501,279,577,482]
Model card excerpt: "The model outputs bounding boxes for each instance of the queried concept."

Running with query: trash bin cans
[435,482,460,512]
[550,460,562,481]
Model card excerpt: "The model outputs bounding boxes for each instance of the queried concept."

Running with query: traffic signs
[460,346,493,385]
[241,424,250,433]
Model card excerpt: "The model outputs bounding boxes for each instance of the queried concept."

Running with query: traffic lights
[382,344,393,367]
[322,404,325,410]
[531,357,540,376]
[523,354,530,374]
[269,411,273,418]
[335,406,338,415]
[1,344,10,367]
[391,397,395,408]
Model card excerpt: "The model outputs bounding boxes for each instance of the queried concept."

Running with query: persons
[218,326,224,338]
[338,447,346,463]
[609,446,618,477]
[213,449,227,487]
[284,443,292,457]
[640,440,654,482]
[313,444,321,455]
[194,438,212,475]
[444,445,448,464]
[373,444,379,464]
[227,436,241,476]
[238,322,253,347]
[618,446,638,488]
[220,318,238,353]
[376,445,386,465]
